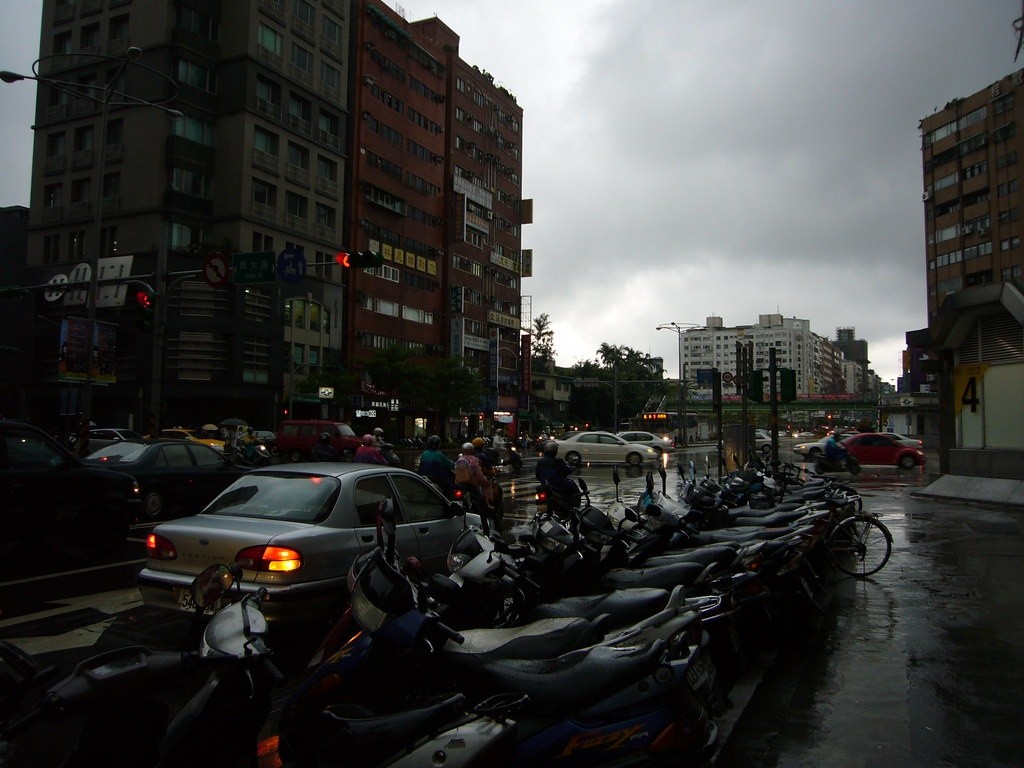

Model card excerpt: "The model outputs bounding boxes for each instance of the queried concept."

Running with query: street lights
[655,322,692,446]
[0,44,187,459]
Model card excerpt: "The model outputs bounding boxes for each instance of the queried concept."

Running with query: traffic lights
[335,249,384,269]
[283,409,288,415]
[136,291,151,308]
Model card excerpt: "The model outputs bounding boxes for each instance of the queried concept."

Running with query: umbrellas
[201,424,218,430]
[79,421,97,427]
[220,418,247,426]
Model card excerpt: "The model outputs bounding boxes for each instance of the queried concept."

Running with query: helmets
[495,429,505,436]
[543,442,558,455]
[472,437,486,449]
[319,433,332,445]
[363,433,373,444]
[462,442,474,455]
[247,427,254,434]
[427,434,441,450]
[373,427,384,436]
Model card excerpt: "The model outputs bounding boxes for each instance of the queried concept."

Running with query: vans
[275,419,363,463]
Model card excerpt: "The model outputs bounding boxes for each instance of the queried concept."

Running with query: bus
[641,411,699,447]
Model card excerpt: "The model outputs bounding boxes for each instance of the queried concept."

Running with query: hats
[255,444,270,458]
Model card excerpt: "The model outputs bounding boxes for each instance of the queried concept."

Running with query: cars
[874,431,924,453]
[87,427,146,454]
[836,432,923,470]
[614,430,676,460]
[543,430,658,467]
[237,430,277,448]
[142,429,227,455]
[755,425,863,460]
[0,418,137,570]
[80,438,256,540]
[134,460,502,638]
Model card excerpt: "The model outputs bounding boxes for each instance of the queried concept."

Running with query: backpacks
[455,453,477,483]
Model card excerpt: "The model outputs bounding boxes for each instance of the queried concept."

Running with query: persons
[824,432,845,464]
[540,431,546,440]
[418,435,455,491]
[78,421,89,456]
[353,434,386,464]
[372,427,385,450]
[221,425,257,447]
[493,428,509,458]
[312,433,336,462]
[535,442,577,490]
[207,430,213,438]
[525,431,529,438]
[472,438,492,470]
[455,442,489,488]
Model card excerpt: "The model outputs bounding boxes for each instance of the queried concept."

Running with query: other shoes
[567,503,577,511]
[481,504,495,510]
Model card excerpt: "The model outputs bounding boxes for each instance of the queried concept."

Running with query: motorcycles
[0,460,895,768]
[812,442,862,476]
[484,440,523,469]
